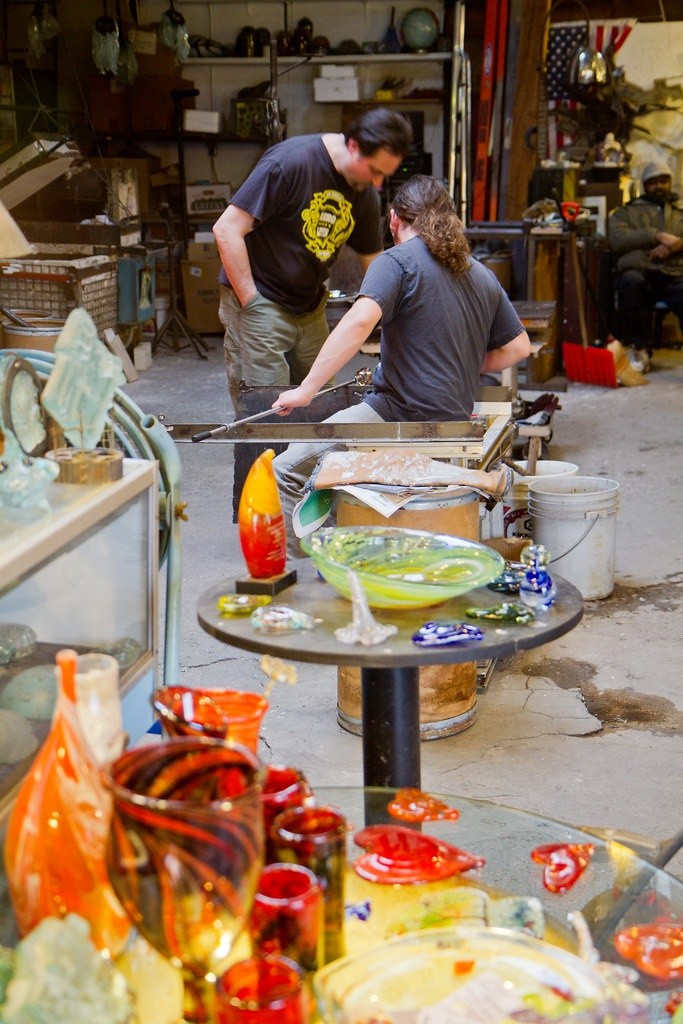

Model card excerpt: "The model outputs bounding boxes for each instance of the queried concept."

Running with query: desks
[2,783,683,1024]
[466,220,571,385]
[197,558,584,829]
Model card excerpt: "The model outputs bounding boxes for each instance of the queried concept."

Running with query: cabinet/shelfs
[0,455,160,828]
[101,53,453,228]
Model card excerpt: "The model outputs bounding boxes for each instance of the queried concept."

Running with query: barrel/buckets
[489,460,578,545]
[526,475,622,601]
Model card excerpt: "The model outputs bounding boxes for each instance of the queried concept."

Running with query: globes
[402,6,440,53]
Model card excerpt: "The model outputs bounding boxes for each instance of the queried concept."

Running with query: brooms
[582,253,651,387]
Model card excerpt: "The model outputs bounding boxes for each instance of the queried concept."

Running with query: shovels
[558,201,618,388]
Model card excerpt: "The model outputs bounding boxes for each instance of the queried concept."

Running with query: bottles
[3,653,124,956]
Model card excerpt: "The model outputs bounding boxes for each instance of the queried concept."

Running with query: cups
[147,682,269,755]
[215,952,304,1024]
[270,806,345,955]
[263,764,315,839]
[249,863,326,972]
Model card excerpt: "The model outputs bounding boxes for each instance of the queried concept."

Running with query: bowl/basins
[313,924,615,1024]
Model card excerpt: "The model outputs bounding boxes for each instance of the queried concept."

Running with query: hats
[642,161,671,183]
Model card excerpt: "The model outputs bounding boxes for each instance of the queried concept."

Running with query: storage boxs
[184,108,223,135]
[180,230,231,334]
[314,65,361,104]
[185,183,233,215]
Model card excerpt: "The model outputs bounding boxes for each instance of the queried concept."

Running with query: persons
[608,162,683,383]
[273,176,530,563]
[212,110,414,419]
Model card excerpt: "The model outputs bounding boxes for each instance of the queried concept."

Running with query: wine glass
[106,736,265,1024]
